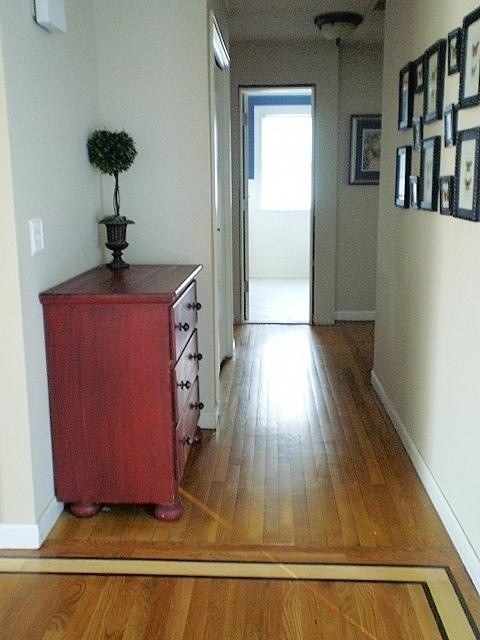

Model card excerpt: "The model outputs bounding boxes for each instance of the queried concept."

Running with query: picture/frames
[392,8,479,224]
[349,113,381,187]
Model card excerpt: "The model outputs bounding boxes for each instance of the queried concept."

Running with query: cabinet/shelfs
[37,261,204,521]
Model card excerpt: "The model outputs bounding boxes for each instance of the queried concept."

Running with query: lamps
[312,11,364,47]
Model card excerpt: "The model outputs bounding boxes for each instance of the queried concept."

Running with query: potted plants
[88,127,138,271]
